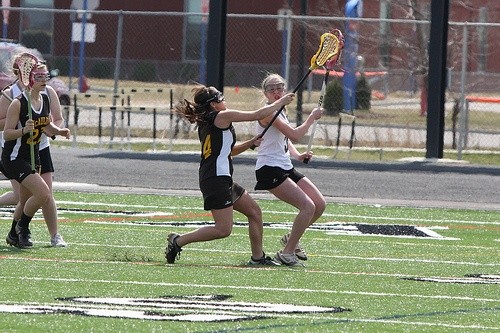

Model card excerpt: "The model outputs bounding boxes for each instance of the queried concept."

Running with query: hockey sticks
[14,52,39,175]
[250,33,341,151]
[303,29,346,164]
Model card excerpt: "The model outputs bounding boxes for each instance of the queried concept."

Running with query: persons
[2,61,72,248]
[0,53,69,248]
[166,85,295,267]
[256,72,329,267]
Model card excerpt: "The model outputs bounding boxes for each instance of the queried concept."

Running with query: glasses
[31,72,51,82]
[265,82,284,94]
[206,92,225,104]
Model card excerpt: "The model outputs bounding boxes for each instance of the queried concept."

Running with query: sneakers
[15,219,34,247]
[280,232,308,261]
[50,233,68,248]
[273,249,306,271]
[164,232,183,264]
[247,251,282,267]
[5,229,19,246]
